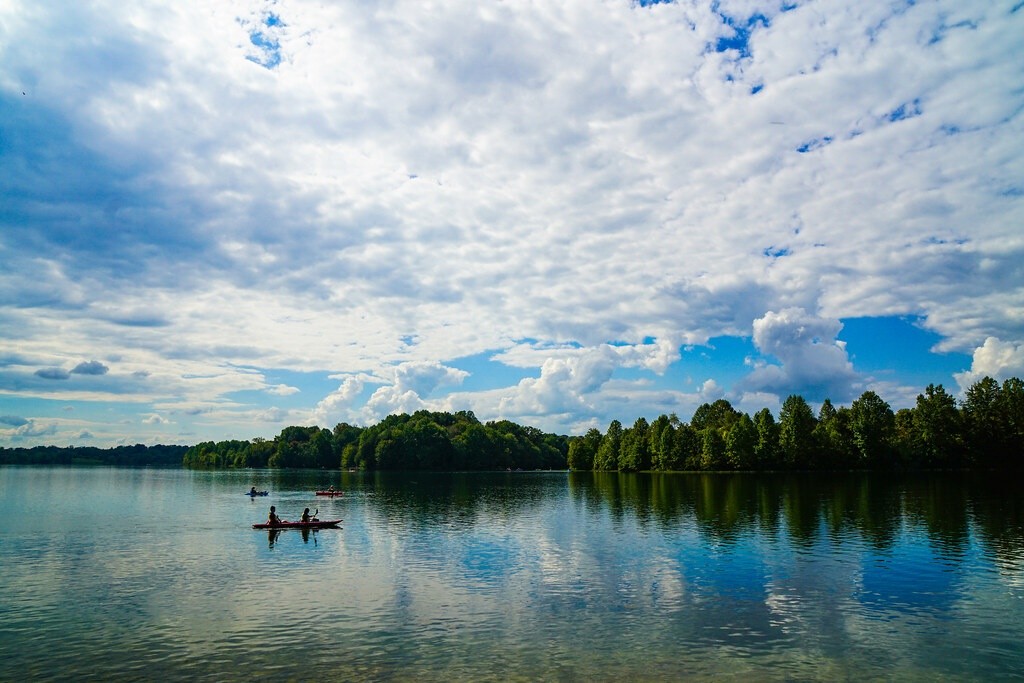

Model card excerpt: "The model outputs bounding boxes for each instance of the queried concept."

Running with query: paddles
[315,508,319,516]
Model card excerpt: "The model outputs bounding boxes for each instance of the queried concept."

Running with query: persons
[250,486,257,493]
[268,506,282,524]
[301,508,315,522]
[330,485,334,491]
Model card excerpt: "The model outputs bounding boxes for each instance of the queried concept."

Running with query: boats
[316,489,342,496]
[244,490,270,496]
[253,519,344,528]
